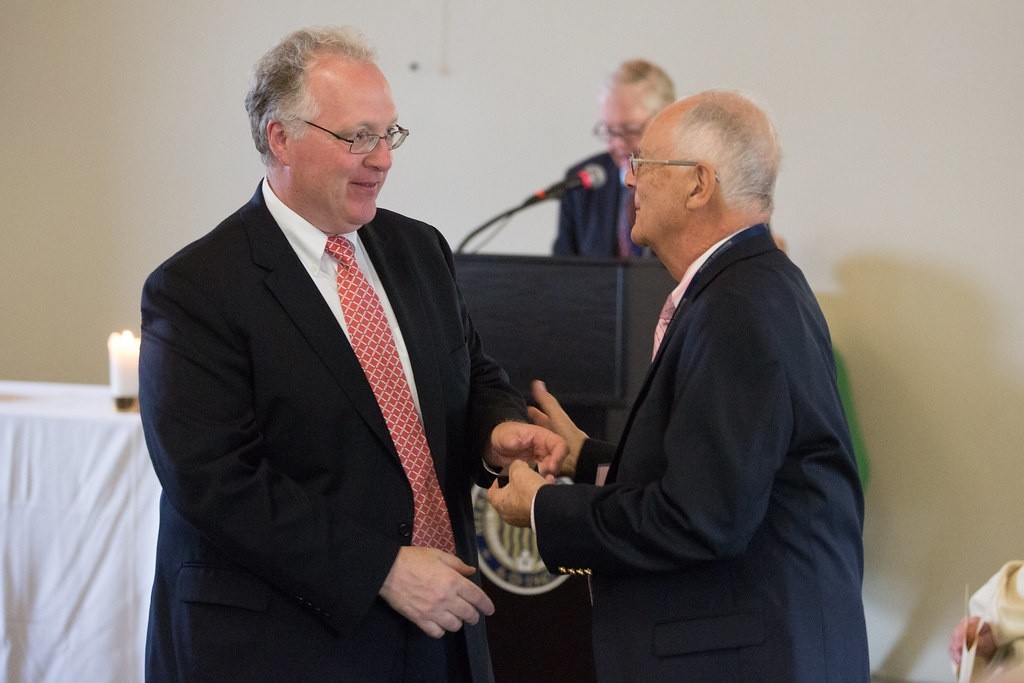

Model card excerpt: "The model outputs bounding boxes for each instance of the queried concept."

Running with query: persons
[949,559,1023,682]
[485,91,870,683]
[553,62,782,262]
[138,25,571,683]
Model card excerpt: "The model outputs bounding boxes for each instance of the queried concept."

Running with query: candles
[106,328,143,414]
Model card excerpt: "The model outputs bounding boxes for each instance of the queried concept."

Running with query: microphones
[457,163,606,253]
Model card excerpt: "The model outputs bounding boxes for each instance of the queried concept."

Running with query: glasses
[629,152,721,184]
[289,114,410,155]
[592,112,654,143]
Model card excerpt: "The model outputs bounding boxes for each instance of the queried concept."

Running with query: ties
[324,235,457,557]
[650,292,677,363]
[617,181,643,257]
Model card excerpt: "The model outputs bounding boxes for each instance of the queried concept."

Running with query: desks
[0,380,163,683]
